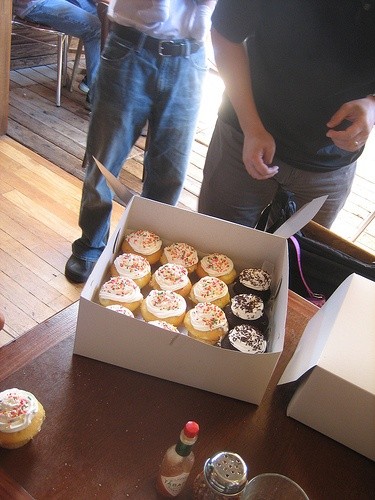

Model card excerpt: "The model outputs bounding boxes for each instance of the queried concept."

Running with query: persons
[65,0,216,282]
[13,0,101,110]
[198,0,375,233]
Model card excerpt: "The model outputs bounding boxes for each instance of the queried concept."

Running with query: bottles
[193,451,248,500]
[155,420,199,498]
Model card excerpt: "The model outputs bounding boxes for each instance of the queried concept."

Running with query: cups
[241,473,310,500]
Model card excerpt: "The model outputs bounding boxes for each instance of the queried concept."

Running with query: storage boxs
[276,272,375,460]
[73,155,328,406]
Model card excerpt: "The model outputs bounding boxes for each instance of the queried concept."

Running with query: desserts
[97,229,272,354]
[0,387,46,449]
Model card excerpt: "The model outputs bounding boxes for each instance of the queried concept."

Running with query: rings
[355,141,359,145]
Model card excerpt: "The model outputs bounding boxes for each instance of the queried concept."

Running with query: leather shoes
[65,255,96,284]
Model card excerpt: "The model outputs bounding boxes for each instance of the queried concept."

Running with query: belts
[110,21,204,59]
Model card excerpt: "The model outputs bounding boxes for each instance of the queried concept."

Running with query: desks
[0,287,375,500]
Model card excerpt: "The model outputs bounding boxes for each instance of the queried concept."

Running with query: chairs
[11,15,86,107]
[263,183,375,301]
